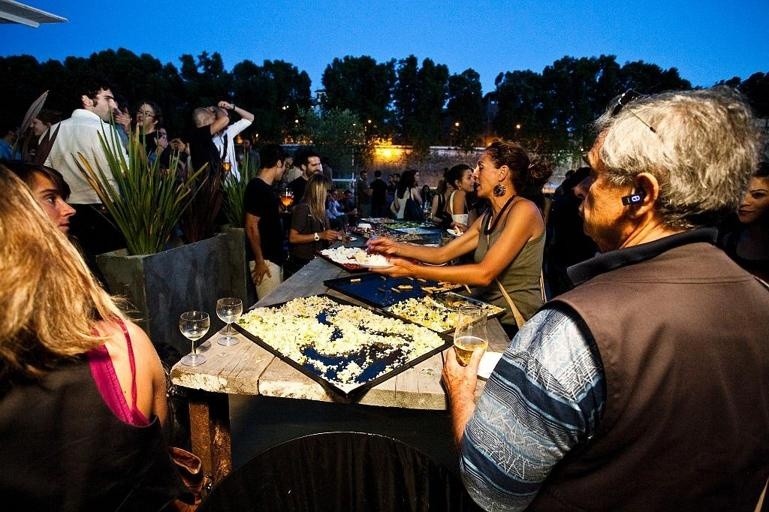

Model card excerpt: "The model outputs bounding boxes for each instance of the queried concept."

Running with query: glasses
[611,88,658,135]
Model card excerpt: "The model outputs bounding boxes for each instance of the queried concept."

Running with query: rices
[232,245,504,394]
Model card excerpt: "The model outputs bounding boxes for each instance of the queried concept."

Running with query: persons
[365,141,548,338]
[2,161,168,512]
[528,163,601,300]
[714,162,767,280]
[357,164,490,239]
[1,74,358,301]
[10,162,77,238]
[440,93,768,512]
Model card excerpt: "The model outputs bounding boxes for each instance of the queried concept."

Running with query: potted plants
[220,153,256,313]
[72,102,229,367]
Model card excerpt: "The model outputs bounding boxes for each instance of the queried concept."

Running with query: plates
[476,352,504,379]
[338,236,356,242]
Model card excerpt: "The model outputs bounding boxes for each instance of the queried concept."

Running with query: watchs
[314,232,320,242]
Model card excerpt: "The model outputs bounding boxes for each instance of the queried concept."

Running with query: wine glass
[179,310,211,368]
[215,297,243,346]
[279,188,293,214]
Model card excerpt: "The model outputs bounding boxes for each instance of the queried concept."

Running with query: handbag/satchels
[404,198,424,221]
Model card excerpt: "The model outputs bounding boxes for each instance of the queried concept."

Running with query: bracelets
[232,104,236,111]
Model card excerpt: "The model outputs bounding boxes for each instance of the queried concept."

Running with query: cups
[223,157,231,171]
[454,304,489,368]
[423,207,432,224]
[341,236,350,246]
[452,214,469,236]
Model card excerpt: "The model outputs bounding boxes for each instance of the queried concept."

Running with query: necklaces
[483,196,516,235]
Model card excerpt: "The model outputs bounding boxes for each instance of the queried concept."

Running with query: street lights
[513,123,521,143]
[450,121,460,146]
[363,119,372,143]
[352,121,357,139]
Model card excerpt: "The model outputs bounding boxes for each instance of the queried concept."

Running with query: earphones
[621,190,646,206]
